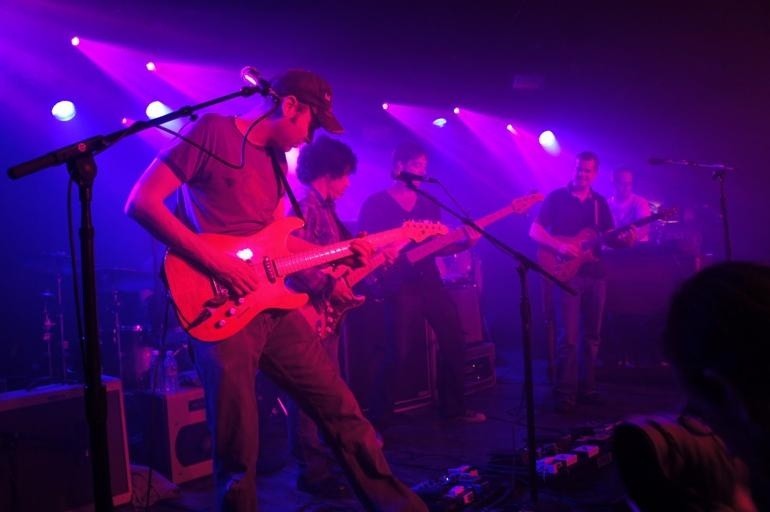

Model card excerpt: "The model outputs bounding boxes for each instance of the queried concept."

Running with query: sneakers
[457,408,488,422]
[554,394,575,413]
[584,387,608,403]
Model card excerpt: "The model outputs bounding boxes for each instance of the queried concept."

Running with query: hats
[273,69,349,135]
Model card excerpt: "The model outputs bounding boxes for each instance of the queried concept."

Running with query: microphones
[390,170,442,193]
[644,154,699,171]
[237,64,283,104]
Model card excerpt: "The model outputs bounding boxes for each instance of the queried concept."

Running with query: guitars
[355,191,545,303]
[289,235,413,337]
[538,205,680,285]
[164,215,449,342]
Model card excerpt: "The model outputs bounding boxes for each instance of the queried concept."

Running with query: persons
[529,152,643,414]
[604,166,652,254]
[605,260,770,512]
[360,140,486,424]
[125,70,429,511]
[288,135,401,500]
